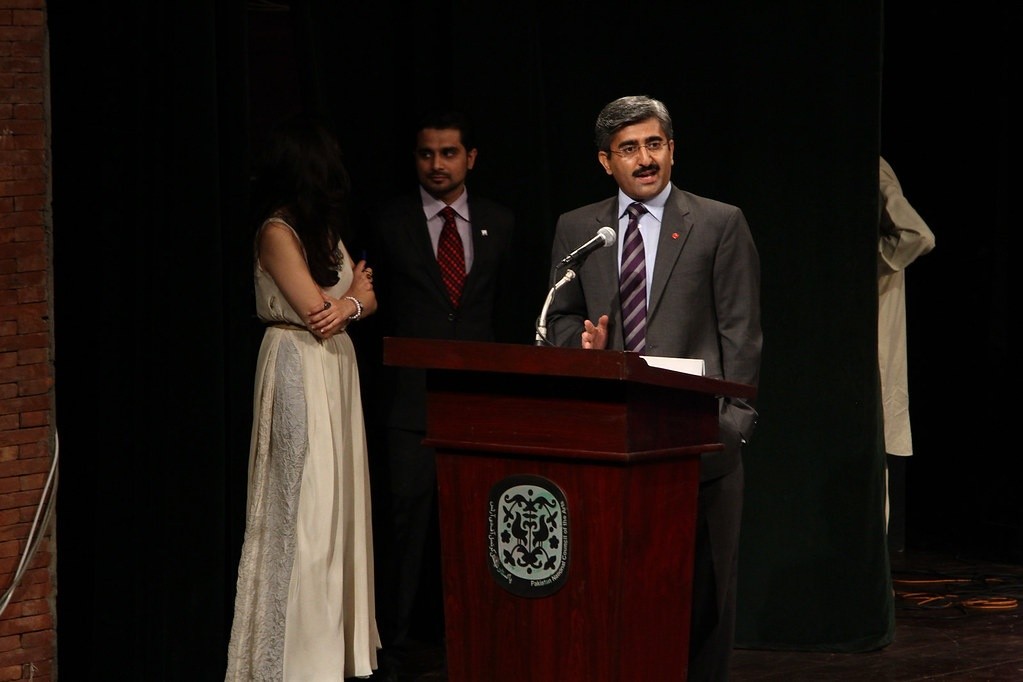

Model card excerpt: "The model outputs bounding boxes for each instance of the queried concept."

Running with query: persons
[535,94,764,682]
[226,109,384,681]
[364,107,516,682]
[877,152,937,573]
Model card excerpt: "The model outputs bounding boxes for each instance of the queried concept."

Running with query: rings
[366,269,372,274]
[323,300,331,309]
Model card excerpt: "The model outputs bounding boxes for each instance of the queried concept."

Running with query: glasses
[610,139,669,157]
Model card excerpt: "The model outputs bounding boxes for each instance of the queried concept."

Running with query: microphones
[556,226,616,269]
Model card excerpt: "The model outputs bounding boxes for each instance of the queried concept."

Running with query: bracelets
[344,296,361,319]
[353,298,363,321]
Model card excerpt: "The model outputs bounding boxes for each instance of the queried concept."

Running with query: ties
[619,203,648,352]
[437,206,467,306]
